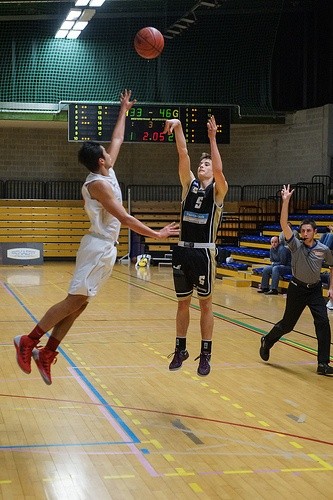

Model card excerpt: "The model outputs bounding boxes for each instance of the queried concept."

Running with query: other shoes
[257,288,269,293]
[326,300,333,310]
[260,337,269,361]
[317,363,333,377]
[264,289,278,295]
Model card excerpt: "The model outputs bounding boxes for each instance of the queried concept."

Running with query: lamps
[163,0,220,39]
[54,0,106,39]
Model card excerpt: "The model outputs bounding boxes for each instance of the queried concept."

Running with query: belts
[292,276,321,289]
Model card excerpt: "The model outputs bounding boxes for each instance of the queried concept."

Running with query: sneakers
[167,348,190,371]
[32,345,59,385]
[13,335,41,374]
[194,351,212,376]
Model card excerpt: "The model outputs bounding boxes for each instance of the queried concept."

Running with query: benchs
[216,200,333,298]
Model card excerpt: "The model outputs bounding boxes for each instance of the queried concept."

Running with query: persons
[256,184,333,376]
[162,115,229,377]
[13,89,182,384]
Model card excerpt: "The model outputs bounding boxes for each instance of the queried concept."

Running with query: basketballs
[134,26,165,59]
